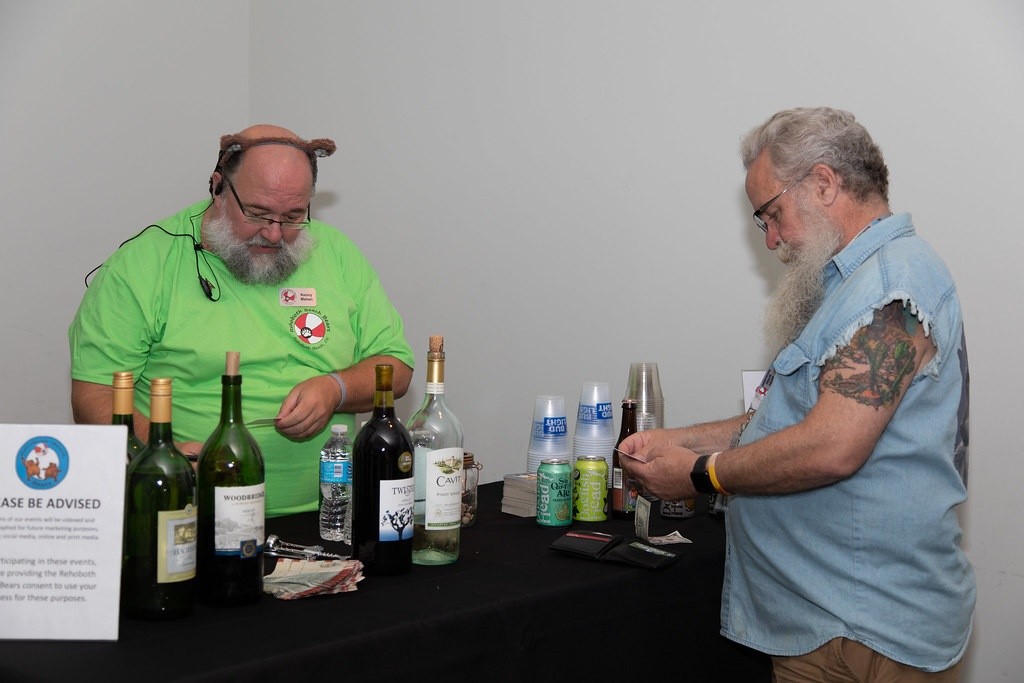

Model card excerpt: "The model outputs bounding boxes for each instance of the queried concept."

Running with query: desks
[0,469,777,683]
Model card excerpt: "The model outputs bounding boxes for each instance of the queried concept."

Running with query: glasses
[753,172,811,233]
[224,176,311,229]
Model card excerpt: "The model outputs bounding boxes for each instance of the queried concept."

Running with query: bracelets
[328,373,347,412]
[708,452,732,495]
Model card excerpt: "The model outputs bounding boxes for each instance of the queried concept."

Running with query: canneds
[536,458,573,526]
[570,455,609,522]
[705,493,726,516]
[659,498,696,518]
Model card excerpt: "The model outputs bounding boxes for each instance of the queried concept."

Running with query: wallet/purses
[549,531,680,571]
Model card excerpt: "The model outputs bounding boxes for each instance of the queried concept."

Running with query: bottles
[110,372,145,467]
[318,420,368,545]
[621,362,665,433]
[406,336,463,565]
[611,398,644,520]
[125,378,197,623]
[197,352,265,605]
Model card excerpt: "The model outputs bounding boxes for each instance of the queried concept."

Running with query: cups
[528,396,571,477]
[573,381,615,489]
[352,365,413,573]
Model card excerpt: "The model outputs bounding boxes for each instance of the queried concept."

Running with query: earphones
[215,181,224,195]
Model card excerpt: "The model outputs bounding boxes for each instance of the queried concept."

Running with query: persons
[71,126,416,519]
[618,107,975,683]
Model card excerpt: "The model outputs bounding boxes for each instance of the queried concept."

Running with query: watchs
[690,455,718,495]
